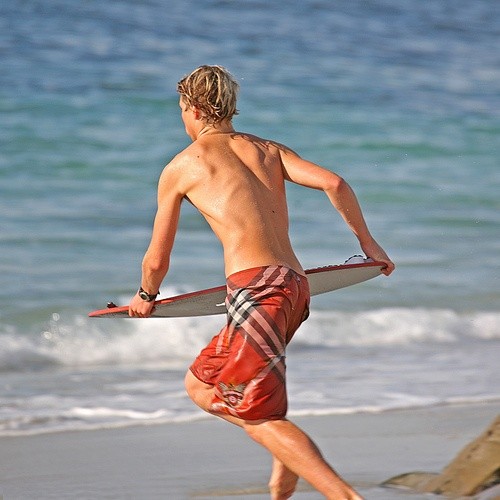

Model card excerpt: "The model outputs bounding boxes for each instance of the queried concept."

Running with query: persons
[126,64,397,500]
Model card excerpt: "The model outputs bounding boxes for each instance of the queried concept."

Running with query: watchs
[139,286,161,302]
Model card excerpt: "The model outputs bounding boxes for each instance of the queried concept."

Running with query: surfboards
[87,256,387,319]
